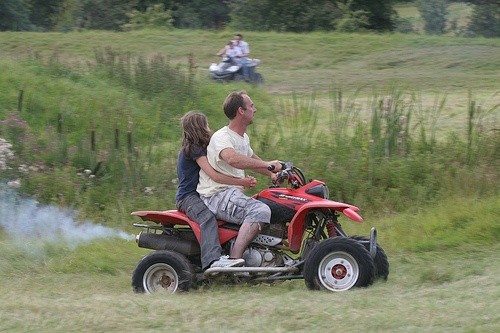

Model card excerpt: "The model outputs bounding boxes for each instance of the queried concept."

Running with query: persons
[216,34,250,82]
[196,90,282,277]
[176,111,245,277]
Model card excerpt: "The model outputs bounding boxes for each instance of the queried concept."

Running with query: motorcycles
[207,53,265,90]
[129,160,390,299]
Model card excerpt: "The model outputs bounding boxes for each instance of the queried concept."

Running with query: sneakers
[230,265,255,277]
[244,79,249,82]
[209,255,245,276]
